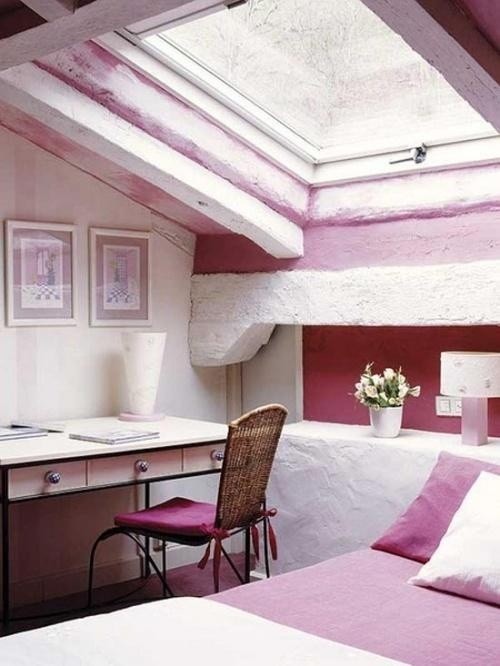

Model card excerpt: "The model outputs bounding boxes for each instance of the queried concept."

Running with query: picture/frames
[5,220,77,326]
[88,227,153,327]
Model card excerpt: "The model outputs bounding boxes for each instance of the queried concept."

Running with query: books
[70,428,160,444]
[0,418,65,441]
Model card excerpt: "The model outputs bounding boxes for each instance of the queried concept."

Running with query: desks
[249,421,500,580]
[0,417,251,636]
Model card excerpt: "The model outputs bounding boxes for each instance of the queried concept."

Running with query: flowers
[351,361,421,409]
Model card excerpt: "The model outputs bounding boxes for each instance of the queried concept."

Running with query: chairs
[87,403,288,612]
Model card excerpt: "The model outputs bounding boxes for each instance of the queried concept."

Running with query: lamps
[440,351,500,446]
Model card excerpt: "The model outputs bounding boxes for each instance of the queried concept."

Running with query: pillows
[369,451,500,564]
[407,470,500,606]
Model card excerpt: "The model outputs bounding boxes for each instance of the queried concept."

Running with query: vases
[369,405,403,437]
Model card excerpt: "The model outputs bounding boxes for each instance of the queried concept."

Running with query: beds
[0,488,500,666]
